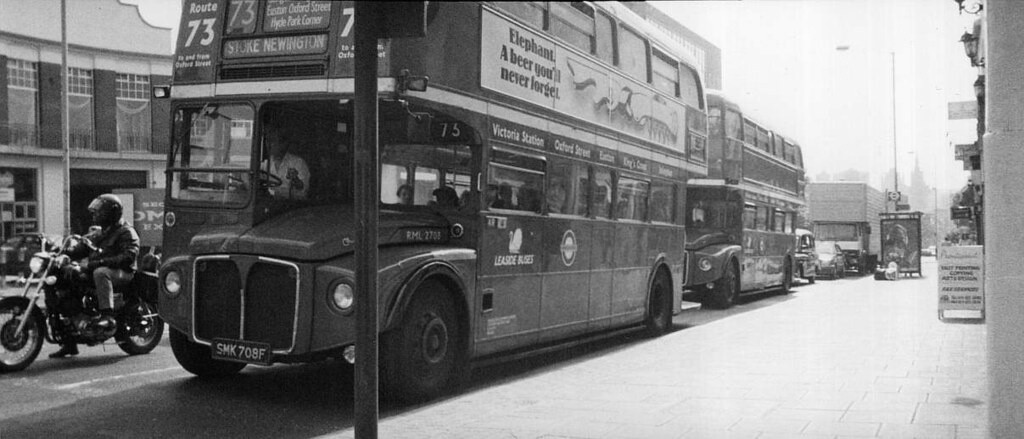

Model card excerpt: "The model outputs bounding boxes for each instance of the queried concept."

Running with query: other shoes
[49,344,78,358]
[94,315,116,328]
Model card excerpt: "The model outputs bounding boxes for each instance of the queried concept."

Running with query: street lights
[833,43,901,214]
[930,186,938,261]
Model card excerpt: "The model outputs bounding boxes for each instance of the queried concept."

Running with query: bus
[812,220,871,278]
[152,0,710,405]
[687,95,806,309]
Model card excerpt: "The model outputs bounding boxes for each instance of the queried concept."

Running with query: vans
[796,228,816,285]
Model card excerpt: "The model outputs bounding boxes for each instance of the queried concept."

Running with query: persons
[884,224,909,269]
[397,176,676,224]
[49,192,141,359]
[230,127,310,198]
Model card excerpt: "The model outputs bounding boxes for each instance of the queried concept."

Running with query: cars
[921,245,936,256]
[815,241,846,280]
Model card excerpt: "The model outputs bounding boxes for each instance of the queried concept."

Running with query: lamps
[957,31,985,68]
[974,79,985,104]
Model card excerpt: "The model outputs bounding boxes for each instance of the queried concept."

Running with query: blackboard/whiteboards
[938,245,983,309]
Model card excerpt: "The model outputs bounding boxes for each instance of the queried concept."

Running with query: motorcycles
[0,235,165,374]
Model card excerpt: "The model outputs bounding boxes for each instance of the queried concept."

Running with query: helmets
[88,193,122,223]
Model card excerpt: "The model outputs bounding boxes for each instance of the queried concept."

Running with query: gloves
[87,260,100,269]
